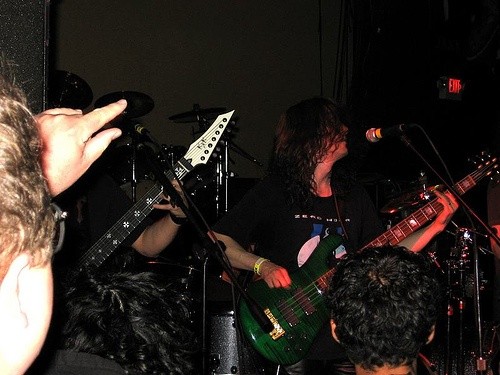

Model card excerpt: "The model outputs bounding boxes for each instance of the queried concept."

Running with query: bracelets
[169,211,185,225]
[253,258,269,276]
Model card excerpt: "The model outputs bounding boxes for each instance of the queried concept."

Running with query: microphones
[364,123,406,143]
[122,112,148,137]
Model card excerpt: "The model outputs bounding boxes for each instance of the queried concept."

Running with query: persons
[1,88,125,374]
[1,95,497,375]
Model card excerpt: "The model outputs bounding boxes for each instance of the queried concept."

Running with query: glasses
[50,203,69,254]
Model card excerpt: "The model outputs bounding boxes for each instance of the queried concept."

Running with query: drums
[161,143,198,189]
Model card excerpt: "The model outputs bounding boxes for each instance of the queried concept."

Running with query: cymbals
[169,106,230,123]
[94,90,155,118]
[44,68,95,108]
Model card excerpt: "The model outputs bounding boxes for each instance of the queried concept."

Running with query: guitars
[63,108,242,289]
[236,150,500,366]
